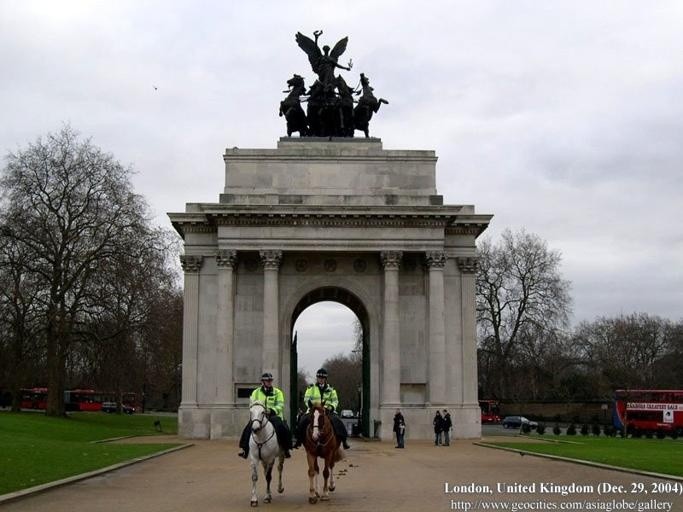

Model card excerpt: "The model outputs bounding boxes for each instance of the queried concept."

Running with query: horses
[248,396,285,507]
[302,398,346,504]
[278,73,389,138]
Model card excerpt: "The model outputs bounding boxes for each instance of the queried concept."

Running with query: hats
[261,373,274,381]
[316,368,328,377]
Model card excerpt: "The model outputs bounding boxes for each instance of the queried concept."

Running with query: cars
[102,402,135,415]
[502,416,538,429]
[340,410,354,418]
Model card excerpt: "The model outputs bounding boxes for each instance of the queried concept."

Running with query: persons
[392,407,404,448]
[313,44,351,90]
[433,410,443,446]
[237,371,294,458]
[290,368,349,451]
[396,418,405,448]
[440,407,451,447]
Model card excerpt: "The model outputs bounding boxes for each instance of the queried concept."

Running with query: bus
[20,387,137,413]
[611,389,683,437]
[479,400,505,424]
